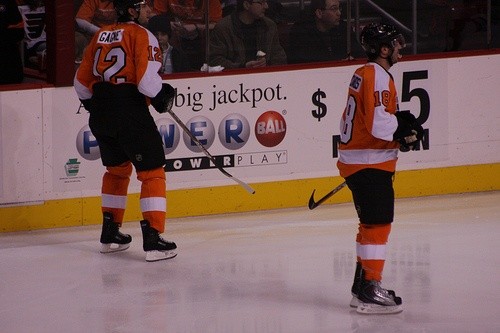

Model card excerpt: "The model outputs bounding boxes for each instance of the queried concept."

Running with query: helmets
[360,23,401,56]
[112,0,147,15]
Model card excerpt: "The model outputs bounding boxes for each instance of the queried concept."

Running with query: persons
[72,0,260,261]
[1,2,367,75]
[335,21,425,315]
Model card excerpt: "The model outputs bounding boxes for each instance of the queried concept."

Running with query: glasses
[249,0,265,6]
[323,7,343,12]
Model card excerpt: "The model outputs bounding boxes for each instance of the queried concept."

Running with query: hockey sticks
[308,180,348,210]
[168,110,255,196]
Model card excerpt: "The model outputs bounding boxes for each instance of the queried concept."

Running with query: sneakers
[348,263,402,314]
[99,211,132,253]
[139,219,177,261]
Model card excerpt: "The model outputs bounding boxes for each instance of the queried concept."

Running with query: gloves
[150,83,175,114]
[394,110,424,151]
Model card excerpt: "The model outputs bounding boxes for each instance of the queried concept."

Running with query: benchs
[0,0,497,86]
